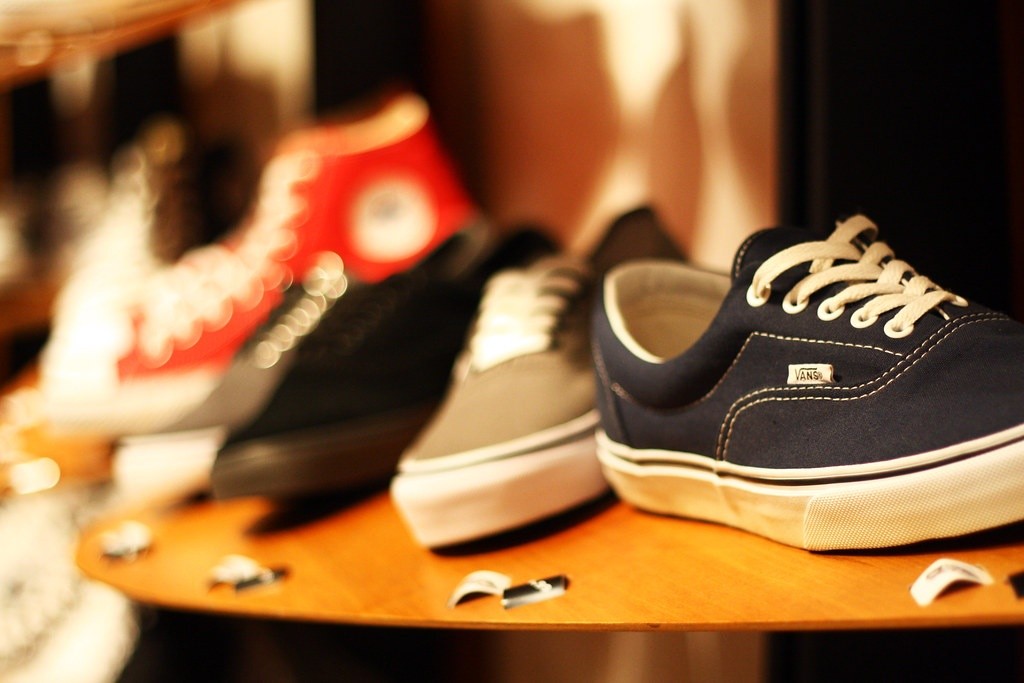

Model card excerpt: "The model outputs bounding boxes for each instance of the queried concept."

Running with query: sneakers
[212,224,561,499]
[388,206,688,550]
[41,94,486,431]
[591,203,1024,552]
